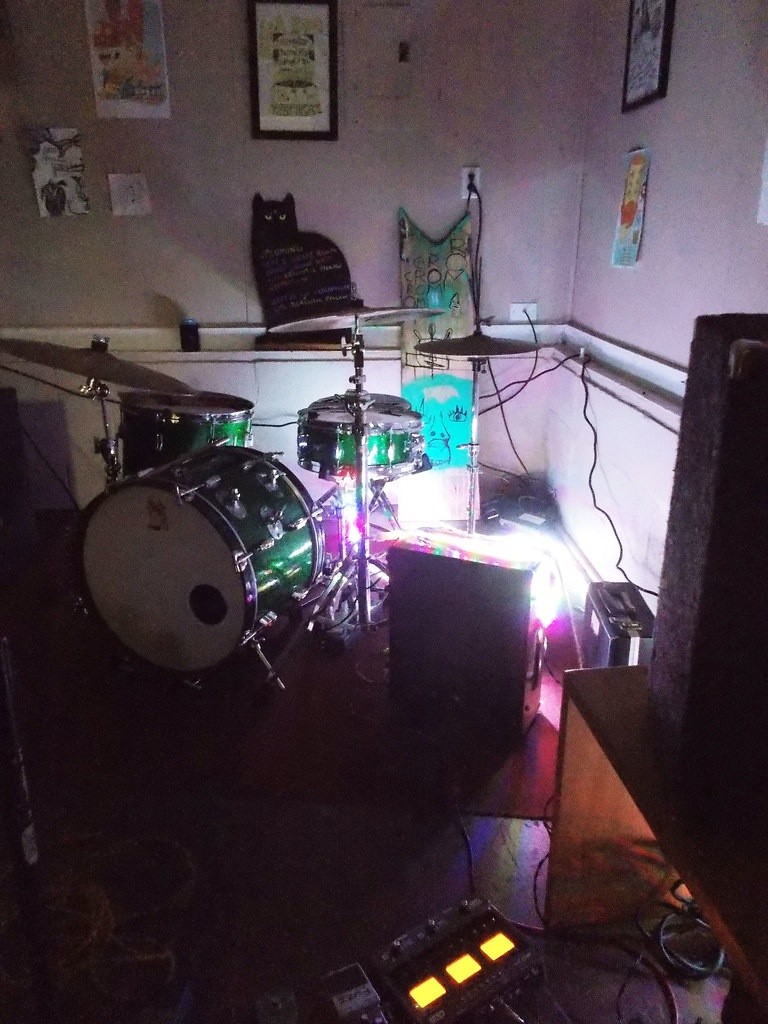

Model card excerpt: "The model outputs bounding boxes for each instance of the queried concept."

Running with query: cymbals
[308,392,413,425]
[0,331,198,393]
[413,332,543,359]
[266,297,448,334]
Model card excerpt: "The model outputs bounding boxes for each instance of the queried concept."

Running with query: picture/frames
[621,0,677,112]
[249,0,339,142]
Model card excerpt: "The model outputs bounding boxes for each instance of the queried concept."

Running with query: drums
[71,442,329,679]
[295,407,424,481]
[117,388,256,481]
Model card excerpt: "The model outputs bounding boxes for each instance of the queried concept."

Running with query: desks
[541,665,768,1024]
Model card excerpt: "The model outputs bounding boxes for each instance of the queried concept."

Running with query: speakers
[389,525,557,734]
[641,312,768,810]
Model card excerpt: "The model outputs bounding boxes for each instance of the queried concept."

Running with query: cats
[250,191,365,331]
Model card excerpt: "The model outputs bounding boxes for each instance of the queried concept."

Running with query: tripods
[251,333,390,700]
